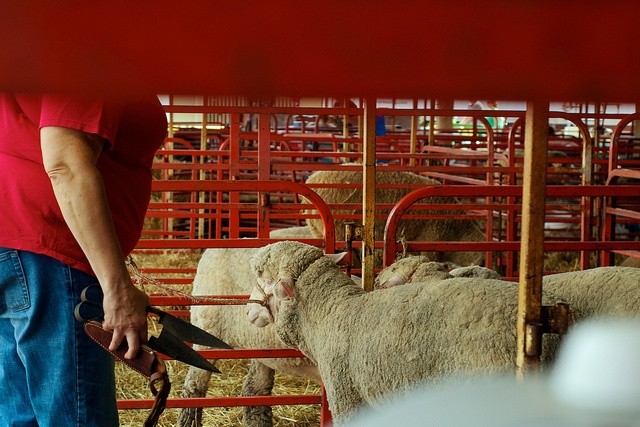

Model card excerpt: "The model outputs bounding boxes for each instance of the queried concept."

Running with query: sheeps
[299,159,511,268]
[176,226,637,427]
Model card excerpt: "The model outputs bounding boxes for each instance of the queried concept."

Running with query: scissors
[74,284,233,374]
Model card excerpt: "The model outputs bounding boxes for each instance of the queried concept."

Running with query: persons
[459,99,483,126]
[484,100,505,130]
[1,95,168,427]
[307,114,339,164]
[334,99,389,167]
